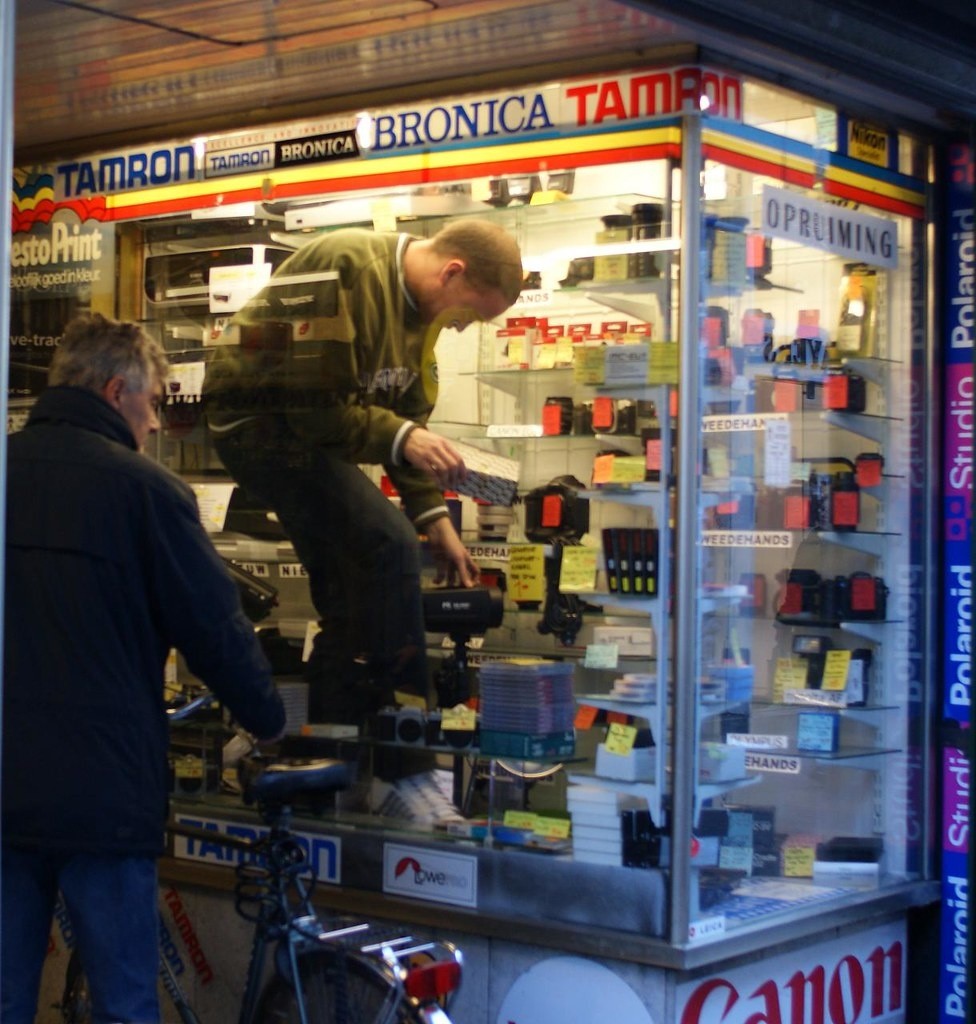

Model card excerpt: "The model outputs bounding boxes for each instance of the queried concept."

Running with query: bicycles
[63,689,464,1024]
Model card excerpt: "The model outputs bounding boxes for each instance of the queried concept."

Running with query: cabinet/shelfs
[9,102,936,974]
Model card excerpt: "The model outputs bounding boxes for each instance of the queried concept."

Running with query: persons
[0,309,300,1024]
[200,216,523,829]
